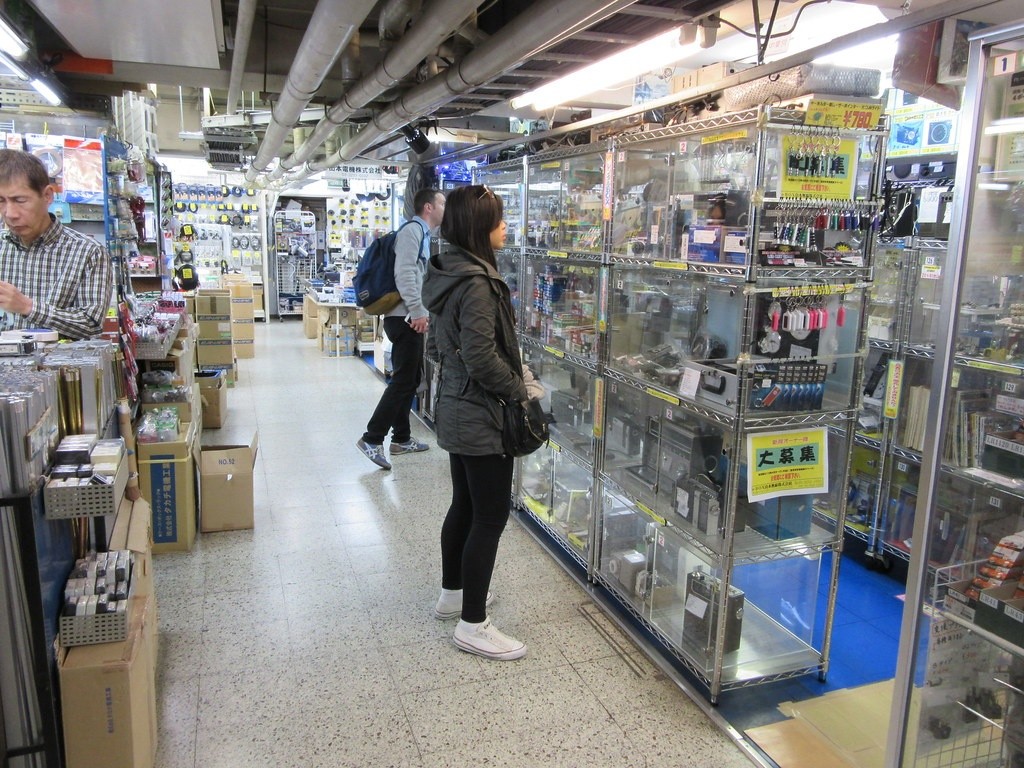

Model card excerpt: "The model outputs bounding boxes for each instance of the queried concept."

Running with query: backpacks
[352,220,425,316]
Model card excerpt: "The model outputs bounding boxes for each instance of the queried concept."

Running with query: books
[890,384,1017,571]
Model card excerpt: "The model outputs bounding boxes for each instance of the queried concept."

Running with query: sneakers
[389,438,430,455]
[453,616,527,661]
[435,591,494,619]
[356,436,392,471]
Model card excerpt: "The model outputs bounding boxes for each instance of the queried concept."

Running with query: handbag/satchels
[500,397,550,458]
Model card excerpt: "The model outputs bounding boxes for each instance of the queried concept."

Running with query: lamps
[0,17,30,61]
[0,52,29,81]
[30,79,61,107]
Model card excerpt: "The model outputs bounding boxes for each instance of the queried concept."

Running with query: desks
[308,294,362,358]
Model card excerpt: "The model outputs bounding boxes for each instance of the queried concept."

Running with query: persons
[421,185,530,661]
[0,149,114,340]
[354,188,447,470]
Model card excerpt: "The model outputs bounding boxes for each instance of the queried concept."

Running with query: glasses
[478,183,494,201]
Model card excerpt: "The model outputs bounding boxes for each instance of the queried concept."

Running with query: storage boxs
[41,272,258,768]
[302,294,376,357]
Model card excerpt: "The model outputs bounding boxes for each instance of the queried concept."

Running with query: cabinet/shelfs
[389,22,1024,768]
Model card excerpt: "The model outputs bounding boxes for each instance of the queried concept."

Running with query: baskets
[42,450,129,519]
[59,556,135,647]
